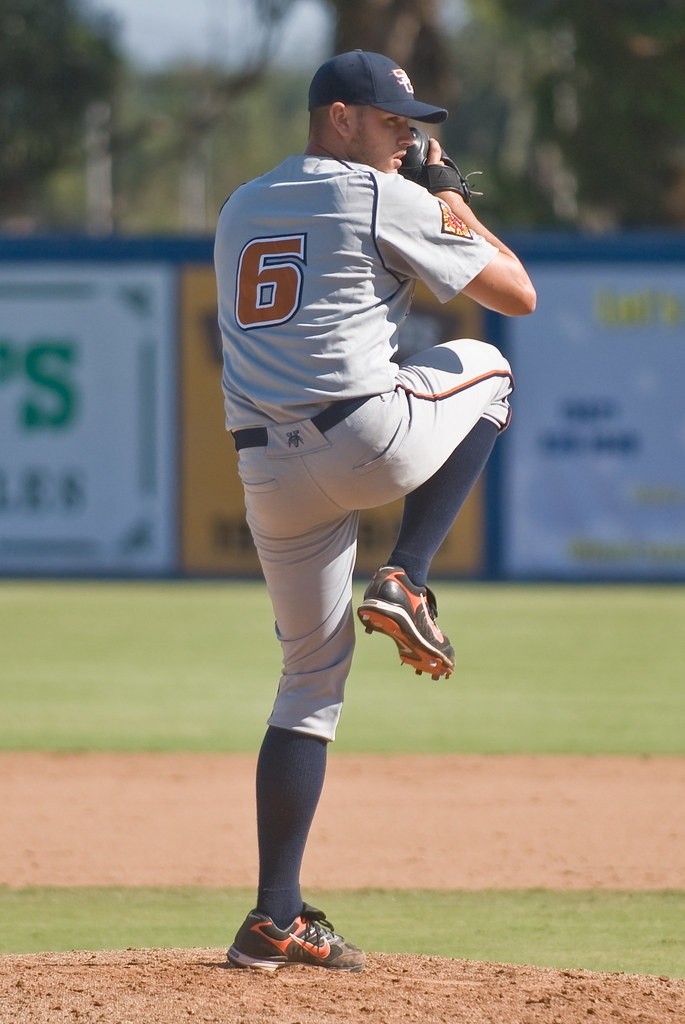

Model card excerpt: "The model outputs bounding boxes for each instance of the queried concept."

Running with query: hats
[308,49,448,123]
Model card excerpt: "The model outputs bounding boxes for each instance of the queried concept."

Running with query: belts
[232,395,375,451]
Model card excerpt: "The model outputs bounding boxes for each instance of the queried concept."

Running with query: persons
[214,48,537,971]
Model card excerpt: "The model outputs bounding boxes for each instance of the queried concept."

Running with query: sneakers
[357,564,455,680]
[227,902,366,973]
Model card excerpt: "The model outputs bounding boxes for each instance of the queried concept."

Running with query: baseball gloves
[398,126,485,206]
